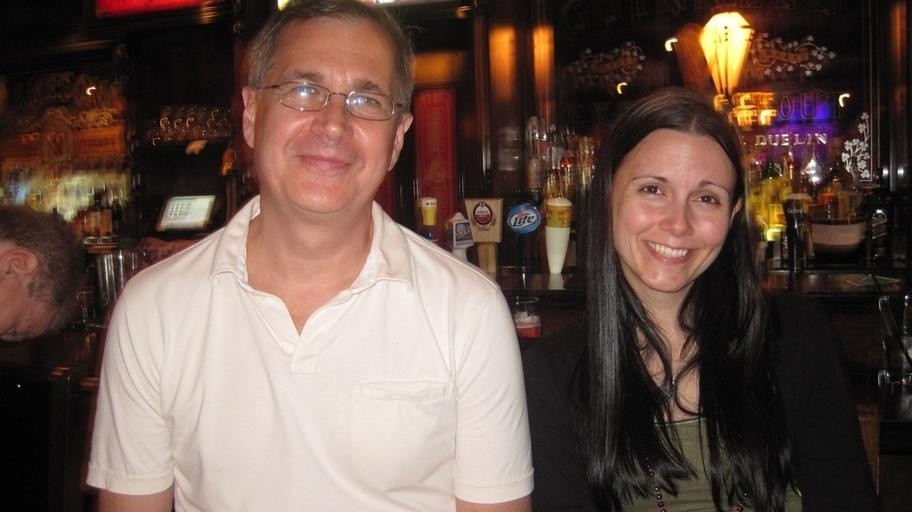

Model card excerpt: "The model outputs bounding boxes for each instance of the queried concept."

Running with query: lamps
[699,8,753,116]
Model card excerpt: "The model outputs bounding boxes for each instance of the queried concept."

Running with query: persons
[523,83,878,512]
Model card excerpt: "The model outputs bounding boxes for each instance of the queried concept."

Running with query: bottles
[739,134,895,275]
[72,187,126,246]
[499,116,598,273]
[887,292,912,379]
[418,197,444,249]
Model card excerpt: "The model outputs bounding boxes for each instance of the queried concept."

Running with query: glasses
[256,81,403,121]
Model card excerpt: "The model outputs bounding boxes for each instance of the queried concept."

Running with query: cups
[506,295,543,339]
